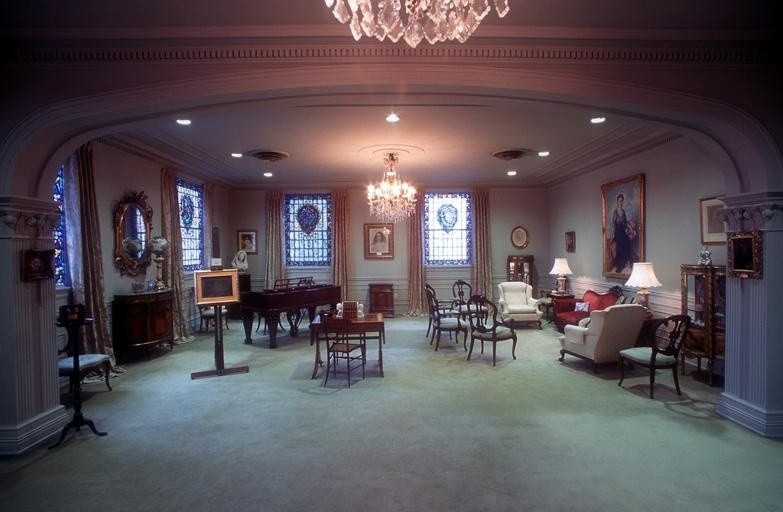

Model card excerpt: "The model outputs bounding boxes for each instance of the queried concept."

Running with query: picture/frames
[193,269,240,304]
[598,172,647,280]
[699,194,728,246]
[236,230,258,255]
[19,247,55,282]
[363,223,395,259]
[726,231,763,280]
[564,231,576,253]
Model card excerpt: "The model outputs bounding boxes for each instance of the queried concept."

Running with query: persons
[230,251,248,271]
[609,192,632,273]
[242,236,250,248]
[371,231,387,252]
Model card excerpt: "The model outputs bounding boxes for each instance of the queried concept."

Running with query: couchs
[552,286,625,330]
[556,303,647,373]
[498,280,544,330]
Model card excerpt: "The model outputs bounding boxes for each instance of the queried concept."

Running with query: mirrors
[112,187,155,275]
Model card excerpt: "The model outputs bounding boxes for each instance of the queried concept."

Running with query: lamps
[548,257,574,293]
[366,152,416,225]
[122,235,141,256]
[546,293,575,323]
[147,235,169,289]
[320,0,511,45]
[628,263,662,316]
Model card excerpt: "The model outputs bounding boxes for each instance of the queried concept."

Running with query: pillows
[575,302,590,313]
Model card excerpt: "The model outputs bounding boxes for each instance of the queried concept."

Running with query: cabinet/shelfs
[112,287,174,364]
[506,254,534,288]
[679,262,724,386]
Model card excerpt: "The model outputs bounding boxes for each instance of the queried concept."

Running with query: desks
[311,311,385,378]
[639,314,668,348]
[237,282,341,349]
[367,283,395,317]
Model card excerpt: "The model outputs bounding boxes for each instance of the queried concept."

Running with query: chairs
[192,286,229,331]
[427,291,468,351]
[423,283,459,340]
[319,313,364,388]
[466,294,517,367]
[617,314,692,398]
[450,278,489,326]
[53,315,112,396]
